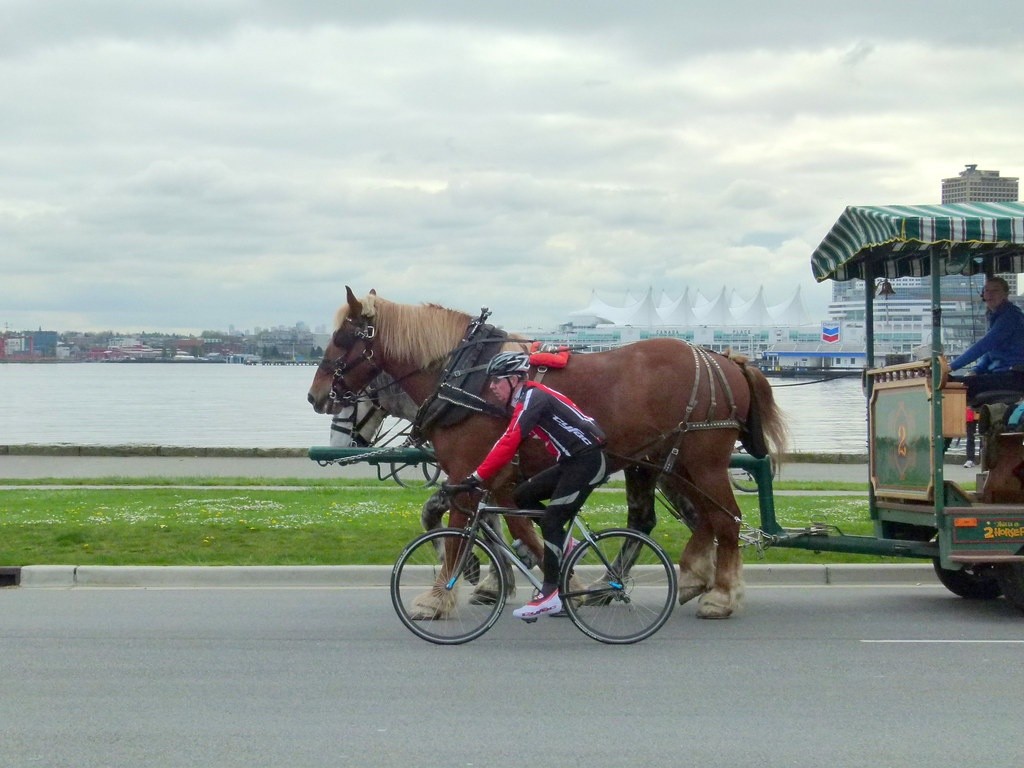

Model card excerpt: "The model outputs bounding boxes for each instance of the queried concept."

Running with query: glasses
[490,374,521,384]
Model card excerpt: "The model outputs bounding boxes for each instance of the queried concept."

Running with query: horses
[307,284,786,623]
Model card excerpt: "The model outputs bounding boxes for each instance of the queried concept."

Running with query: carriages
[308,198,1024,614]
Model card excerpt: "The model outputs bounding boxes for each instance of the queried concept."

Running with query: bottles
[510,538,539,569]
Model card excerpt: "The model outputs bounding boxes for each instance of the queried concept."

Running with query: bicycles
[391,477,677,646]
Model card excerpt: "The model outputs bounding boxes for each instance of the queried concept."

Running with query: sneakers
[563,537,587,564]
[513,586,562,619]
[963,460,975,467]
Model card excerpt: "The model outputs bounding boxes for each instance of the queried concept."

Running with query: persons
[459,350,609,618]
[947,277,1024,399]
[943,422,977,468]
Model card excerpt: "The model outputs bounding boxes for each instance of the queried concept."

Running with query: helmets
[485,351,530,374]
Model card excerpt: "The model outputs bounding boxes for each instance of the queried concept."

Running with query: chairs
[972,388,1024,405]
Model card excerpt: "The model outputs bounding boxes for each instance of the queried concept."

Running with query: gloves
[461,474,481,487]
